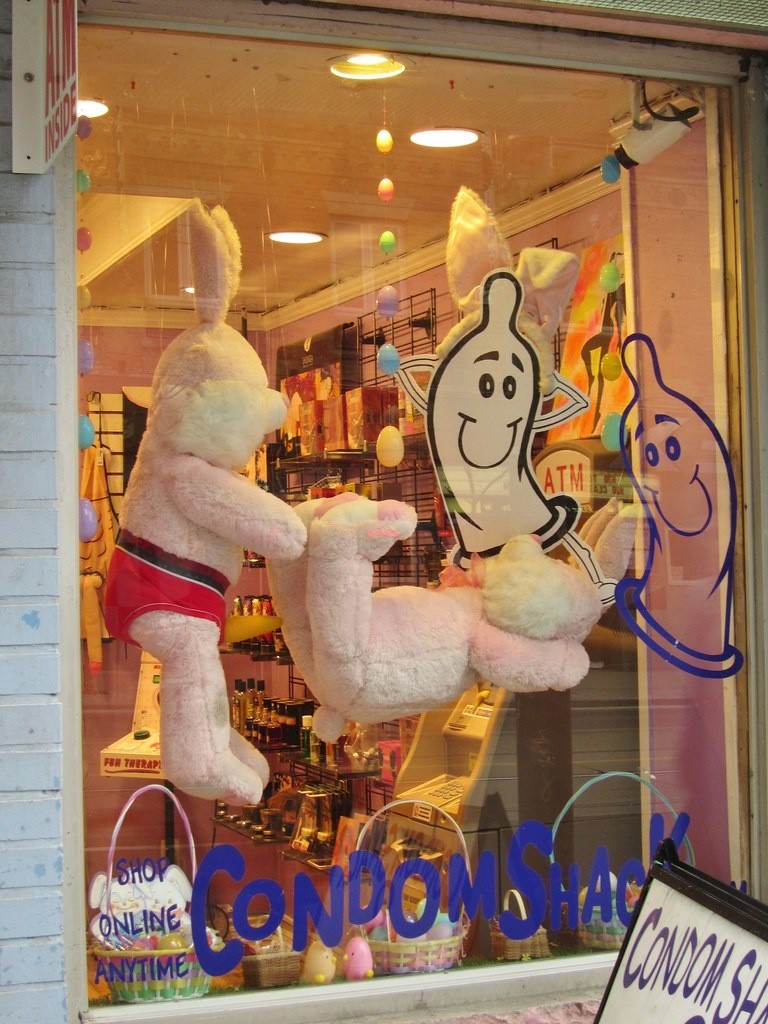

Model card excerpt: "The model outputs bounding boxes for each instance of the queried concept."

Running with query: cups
[261,809,282,830]
[242,803,264,824]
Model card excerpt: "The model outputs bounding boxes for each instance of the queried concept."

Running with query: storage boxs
[240,914,302,986]
[489,889,550,960]
[298,385,399,463]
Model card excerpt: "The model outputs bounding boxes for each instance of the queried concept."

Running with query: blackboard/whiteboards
[595,878,767,1024]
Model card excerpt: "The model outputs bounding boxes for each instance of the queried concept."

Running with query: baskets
[577,906,634,949]
[206,913,347,989]
[356,799,471,974]
[93,784,226,1003]
[490,889,549,960]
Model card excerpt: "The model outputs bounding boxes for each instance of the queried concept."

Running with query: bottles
[232,594,285,654]
[231,678,336,764]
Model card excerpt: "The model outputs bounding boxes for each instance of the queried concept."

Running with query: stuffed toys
[435,184,581,394]
[268,491,640,743]
[106,197,307,806]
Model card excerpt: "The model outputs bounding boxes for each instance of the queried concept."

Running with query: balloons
[78,166,99,545]
[375,129,406,468]
[156,933,186,951]
[599,156,629,452]
[360,898,458,944]
